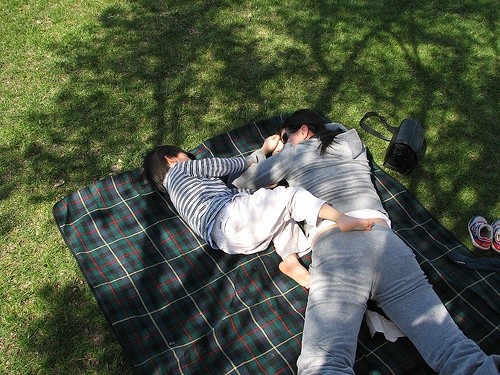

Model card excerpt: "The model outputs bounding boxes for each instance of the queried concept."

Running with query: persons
[143,134,372,289]
[229,110,500,375]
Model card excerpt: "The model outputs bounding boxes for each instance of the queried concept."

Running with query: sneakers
[468,215,493,250]
[491,219,500,253]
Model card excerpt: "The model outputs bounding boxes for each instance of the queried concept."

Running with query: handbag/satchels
[360,112,424,175]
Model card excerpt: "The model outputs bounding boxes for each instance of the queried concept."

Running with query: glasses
[282,128,299,144]
